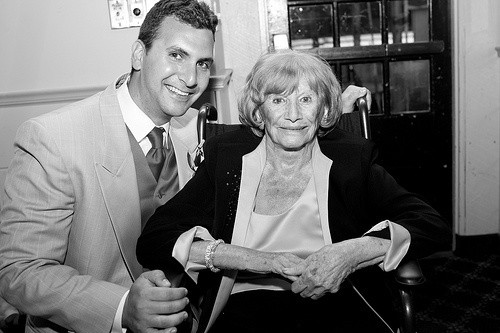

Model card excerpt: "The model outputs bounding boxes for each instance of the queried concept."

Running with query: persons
[0,0,371,333]
[135,51,440,333]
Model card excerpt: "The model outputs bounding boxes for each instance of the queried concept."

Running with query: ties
[146,127,168,182]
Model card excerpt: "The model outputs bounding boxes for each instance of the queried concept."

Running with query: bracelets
[205,239,225,274]
